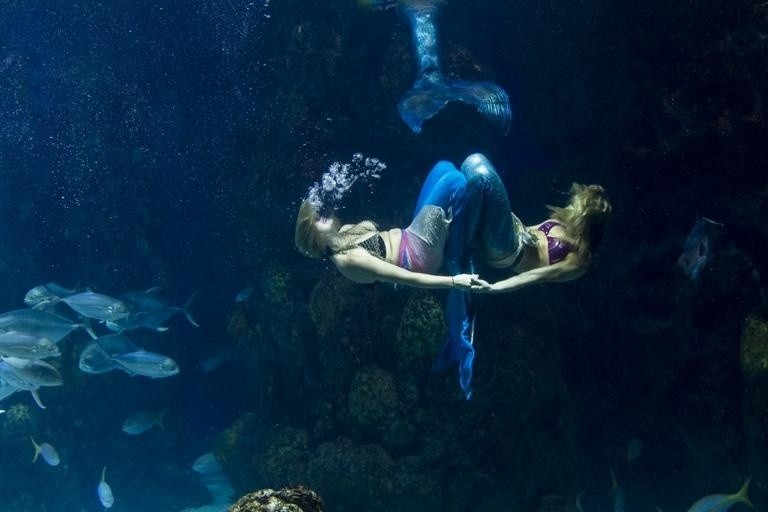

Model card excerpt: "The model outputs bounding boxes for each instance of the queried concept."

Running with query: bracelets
[450,276,456,290]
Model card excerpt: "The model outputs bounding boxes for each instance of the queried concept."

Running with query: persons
[461,152,612,296]
[294,160,479,293]
